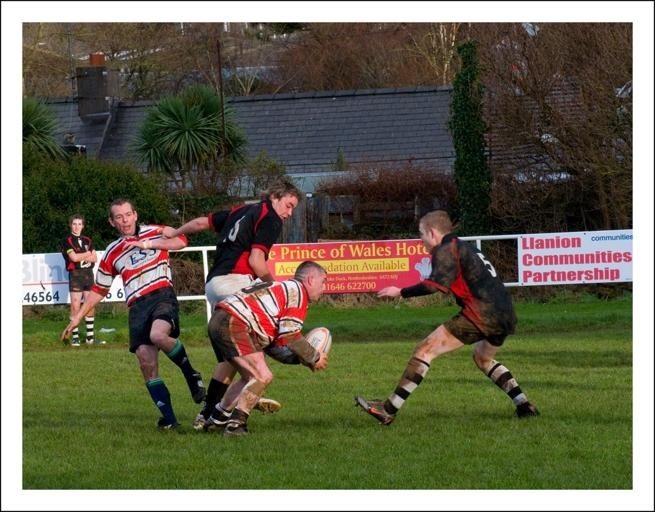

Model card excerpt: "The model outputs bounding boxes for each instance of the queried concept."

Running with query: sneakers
[513,404,541,418]
[86,336,108,345]
[186,369,206,405]
[354,395,397,426]
[156,417,183,430]
[192,398,282,437]
[72,336,81,347]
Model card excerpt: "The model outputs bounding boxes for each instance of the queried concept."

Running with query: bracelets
[144,240,153,250]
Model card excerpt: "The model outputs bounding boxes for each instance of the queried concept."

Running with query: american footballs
[302,328,332,364]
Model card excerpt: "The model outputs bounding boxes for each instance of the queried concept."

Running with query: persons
[157,181,304,431]
[354,210,540,426]
[206,261,330,437]
[62,214,97,346]
[62,198,206,430]
